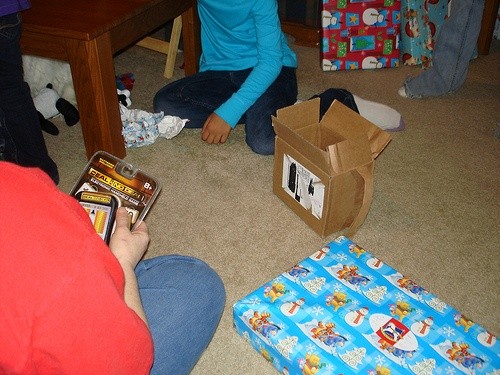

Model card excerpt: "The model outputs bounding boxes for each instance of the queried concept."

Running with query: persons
[0,158,226,375]
[153,0,405,155]
[399,0,485,102]
[0,0,60,186]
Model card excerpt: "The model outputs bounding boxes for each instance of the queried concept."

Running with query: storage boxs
[401,1,479,67]
[319,0,401,71]
[233,235,500,375]
[271,98,392,239]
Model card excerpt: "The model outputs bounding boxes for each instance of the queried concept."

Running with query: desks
[20,0,202,163]
